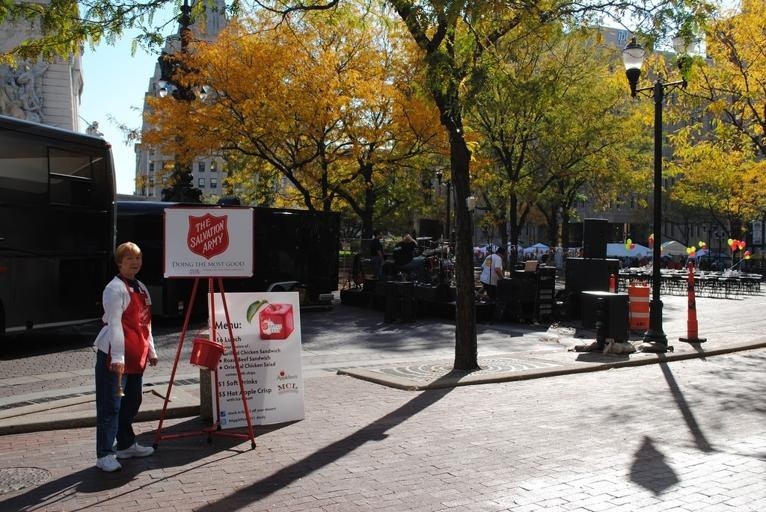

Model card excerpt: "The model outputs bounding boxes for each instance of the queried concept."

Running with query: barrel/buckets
[190,328,226,371]
[595,297,628,344]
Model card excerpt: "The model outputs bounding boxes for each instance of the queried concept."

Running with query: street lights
[436,168,452,240]
[158,1,210,201]
[621,36,693,342]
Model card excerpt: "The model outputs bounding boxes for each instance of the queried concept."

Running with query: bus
[1,115,341,327]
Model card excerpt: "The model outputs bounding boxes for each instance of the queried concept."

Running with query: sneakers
[96,454,122,472]
[115,443,155,458]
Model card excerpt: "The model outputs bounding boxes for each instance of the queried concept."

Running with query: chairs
[494,279,525,322]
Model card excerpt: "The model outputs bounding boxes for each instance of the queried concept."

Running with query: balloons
[691,247,696,252]
[627,239,632,244]
[744,250,750,256]
[626,244,631,249]
[699,241,703,247]
[690,252,696,257]
[702,246,707,250]
[744,255,751,260]
[727,238,746,252]
[687,248,692,253]
[703,242,706,246]
[631,243,635,249]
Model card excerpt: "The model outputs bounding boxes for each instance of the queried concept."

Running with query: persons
[370,230,386,276]
[391,232,419,264]
[92,241,160,474]
[615,256,687,271]
[564,247,585,258]
[479,247,508,302]
[518,249,554,264]
